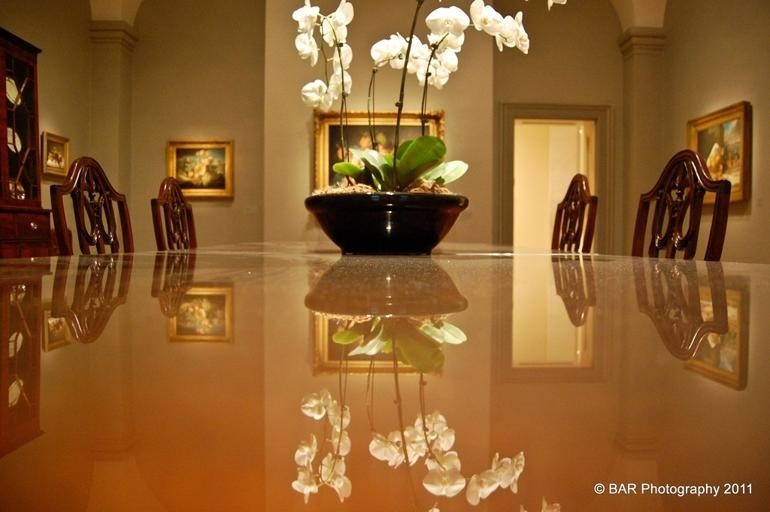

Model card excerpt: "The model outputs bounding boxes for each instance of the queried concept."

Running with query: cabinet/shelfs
[0,25,55,259]
[0,269,48,459]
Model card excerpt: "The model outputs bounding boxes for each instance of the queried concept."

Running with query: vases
[303,185,470,257]
[305,257,470,319]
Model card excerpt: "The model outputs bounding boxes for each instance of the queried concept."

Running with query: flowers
[292,0,568,195]
[290,319,562,512]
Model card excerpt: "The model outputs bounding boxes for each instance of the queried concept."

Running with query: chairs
[50,156,135,256]
[51,255,135,345]
[630,150,732,262]
[551,254,597,327]
[150,176,198,251]
[631,257,729,360]
[151,251,197,318]
[550,173,599,254]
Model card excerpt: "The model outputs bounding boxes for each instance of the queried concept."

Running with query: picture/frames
[313,107,447,230]
[41,131,71,183]
[685,101,753,208]
[41,307,72,351]
[165,140,235,201]
[166,283,235,345]
[679,274,750,391]
